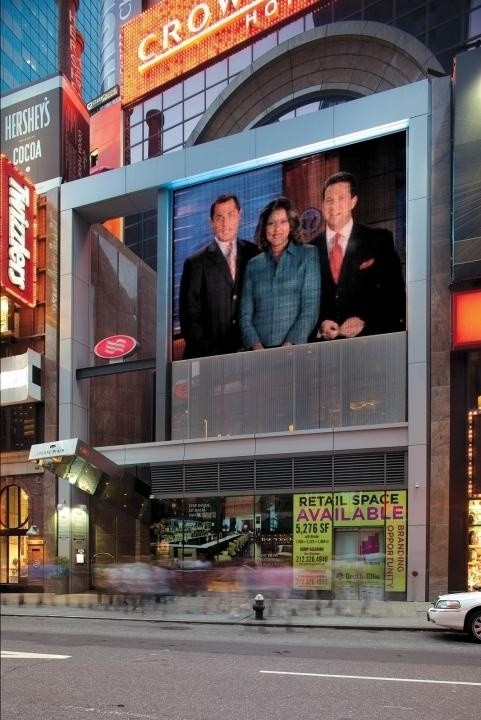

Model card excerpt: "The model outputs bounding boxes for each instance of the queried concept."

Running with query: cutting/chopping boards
[252,593,266,620]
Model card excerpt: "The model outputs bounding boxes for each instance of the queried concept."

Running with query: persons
[179,194,260,358]
[309,171,406,343]
[239,195,322,349]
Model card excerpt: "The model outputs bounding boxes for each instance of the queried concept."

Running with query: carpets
[330,232,344,284]
[227,241,237,280]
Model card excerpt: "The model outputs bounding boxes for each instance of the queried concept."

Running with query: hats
[426,591,481,642]
[270,545,294,557]
